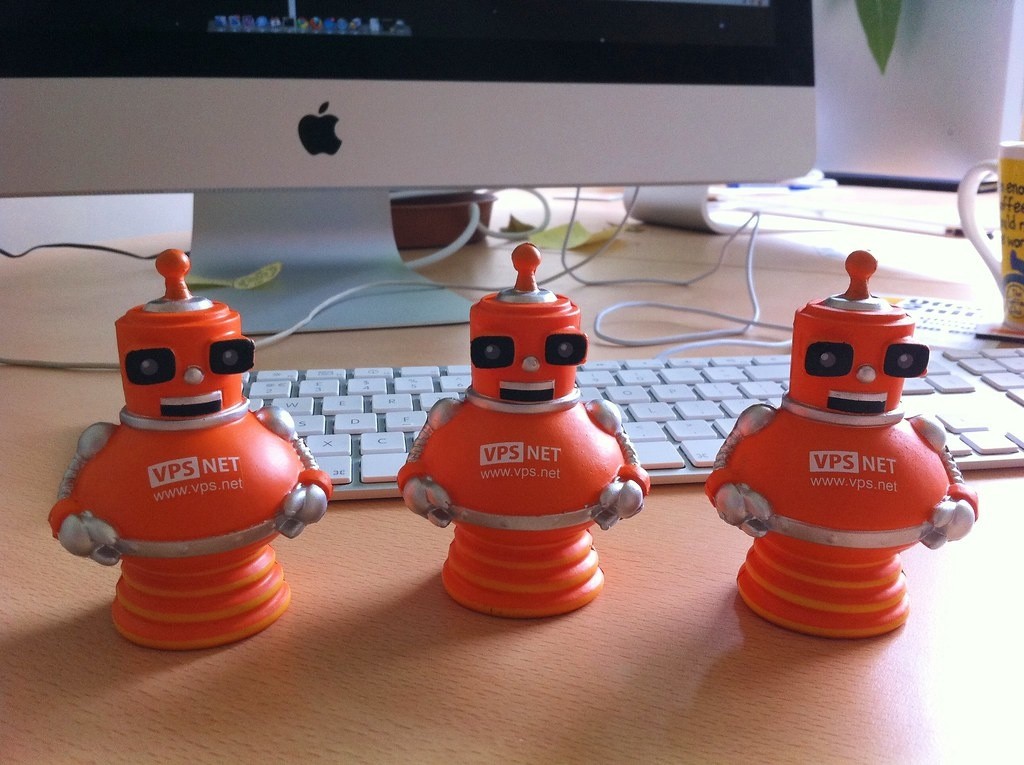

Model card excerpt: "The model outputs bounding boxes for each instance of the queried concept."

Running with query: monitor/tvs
[0,0,818,336]
[625,0,1024,236]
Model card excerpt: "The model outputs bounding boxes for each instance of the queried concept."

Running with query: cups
[956,139,1024,335]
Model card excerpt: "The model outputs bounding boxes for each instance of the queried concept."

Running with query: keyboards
[240,345,1024,503]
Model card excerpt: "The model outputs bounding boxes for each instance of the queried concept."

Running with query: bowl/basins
[388,192,498,249]
